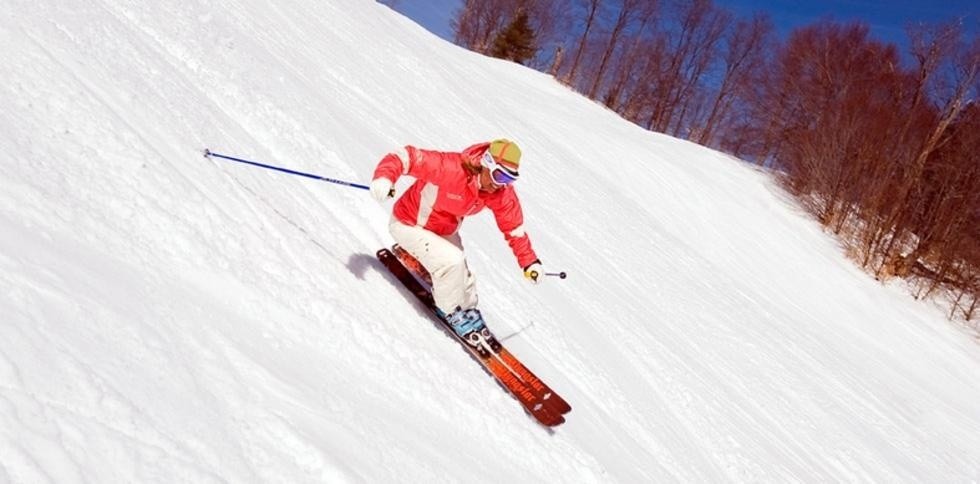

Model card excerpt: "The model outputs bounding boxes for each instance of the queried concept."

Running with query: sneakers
[437,306,489,340]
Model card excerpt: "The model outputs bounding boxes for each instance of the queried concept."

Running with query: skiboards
[377,244,571,426]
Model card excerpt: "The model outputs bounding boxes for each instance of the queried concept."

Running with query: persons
[368,138,547,351]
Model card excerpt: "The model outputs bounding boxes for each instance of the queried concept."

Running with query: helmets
[480,140,521,170]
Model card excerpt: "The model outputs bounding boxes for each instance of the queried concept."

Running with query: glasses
[482,151,519,186]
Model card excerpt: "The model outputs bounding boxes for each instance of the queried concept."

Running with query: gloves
[526,262,543,284]
[370,178,392,202]
